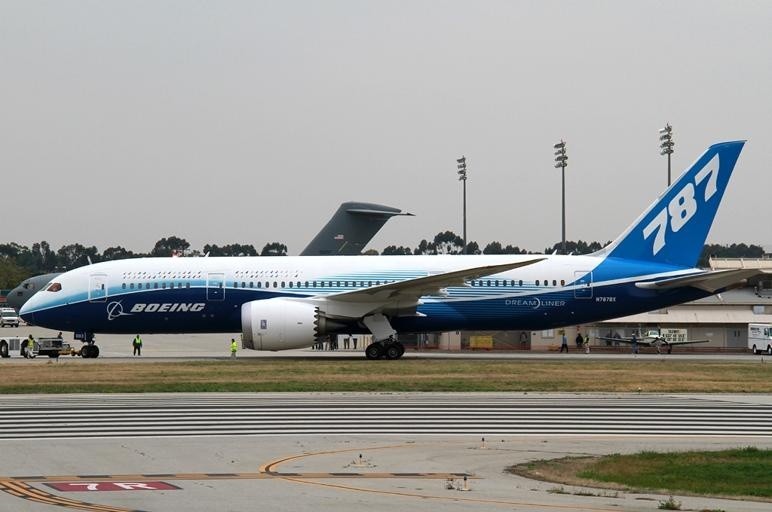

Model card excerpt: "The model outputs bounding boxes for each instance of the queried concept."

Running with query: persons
[132,334,143,356]
[24,334,36,358]
[58,332,63,338]
[311,333,357,352]
[424,334,429,348]
[519,332,528,351]
[230,339,237,360]
[559,330,662,355]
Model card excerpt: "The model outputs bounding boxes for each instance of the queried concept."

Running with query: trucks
[747,322,772,356]
[0,307,20,328]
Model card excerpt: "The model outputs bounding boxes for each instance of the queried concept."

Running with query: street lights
[661,123,674,187]
[456,155,468,244]
[553,139,569,252]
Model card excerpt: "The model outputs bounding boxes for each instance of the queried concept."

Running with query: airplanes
[5,272,65,314]
[300,200,416,255]
[19,139,772,360]
[594,325,711,354]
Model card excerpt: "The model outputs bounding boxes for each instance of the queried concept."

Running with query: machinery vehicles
[0,337,71,358]
